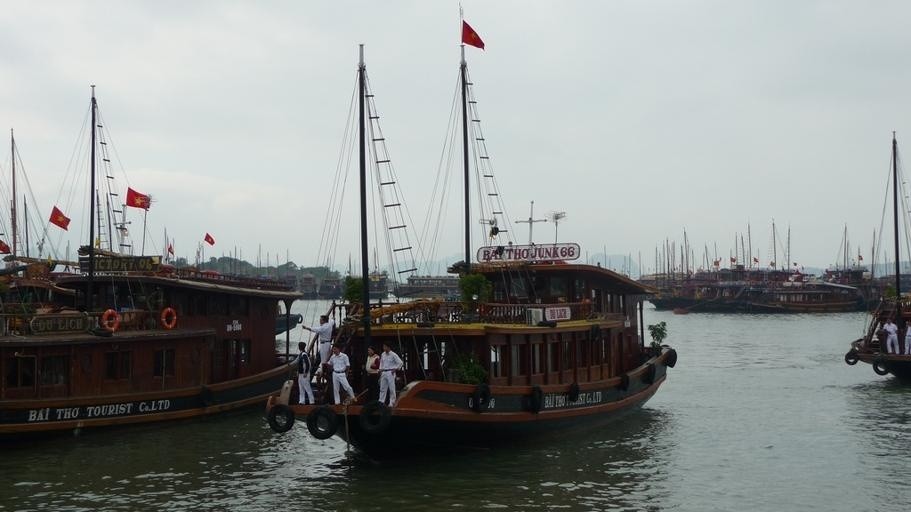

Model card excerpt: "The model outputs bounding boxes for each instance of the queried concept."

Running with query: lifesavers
[103,309,120,332]
[161,307,176,329]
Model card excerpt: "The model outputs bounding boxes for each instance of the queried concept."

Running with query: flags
[1,239,12,253]
[125,189,150,210]
[168,244,175,254]
[50,207,70,229]
[204,231,215,247]
[462,21,486,49]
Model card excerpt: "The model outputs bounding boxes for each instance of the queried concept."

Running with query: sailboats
[842,132,910,381]
[1,86,312,438]
[624,219,910,313]
[265,3,679,456]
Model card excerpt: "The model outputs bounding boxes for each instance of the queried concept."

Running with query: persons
[301,314,335,372]
[377,343,404,408]
[904,319,911,356]
[364,346,379,400]
[326,345,358,407]
[882,319,900,354]
[297,341,316,405]
